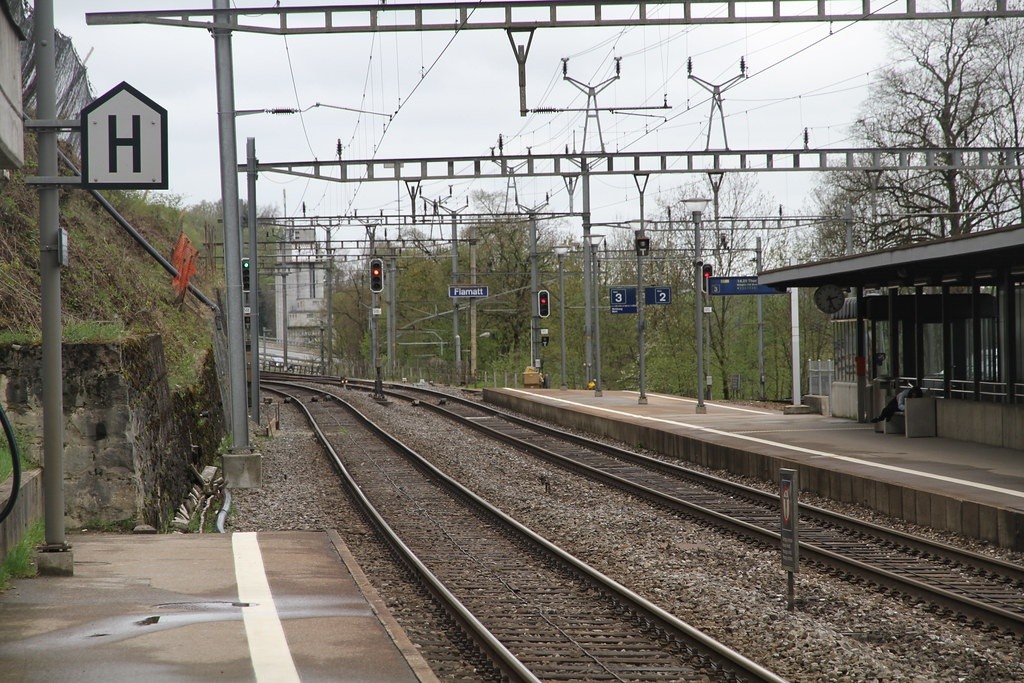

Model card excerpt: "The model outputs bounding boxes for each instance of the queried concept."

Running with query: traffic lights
[368,258,384,294]
[538,290,550,319]
[701,264,714,294]
[242,257,250,293]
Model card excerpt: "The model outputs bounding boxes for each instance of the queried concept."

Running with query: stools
[883,396,936,438]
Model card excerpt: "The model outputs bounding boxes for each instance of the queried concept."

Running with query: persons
[870,386,923,423]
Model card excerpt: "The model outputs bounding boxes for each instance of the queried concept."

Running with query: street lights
[552,245,573,392]
[681,197,714,416]
